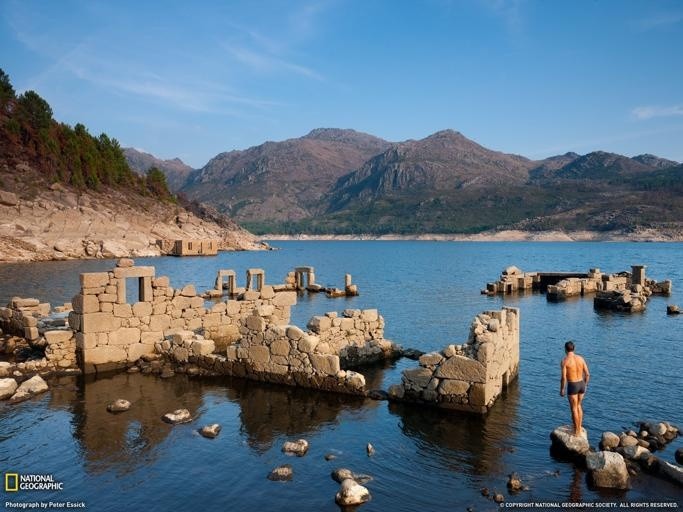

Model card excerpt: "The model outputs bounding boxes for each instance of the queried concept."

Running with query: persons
[559,340,590,437]
[569,464,590,509]
